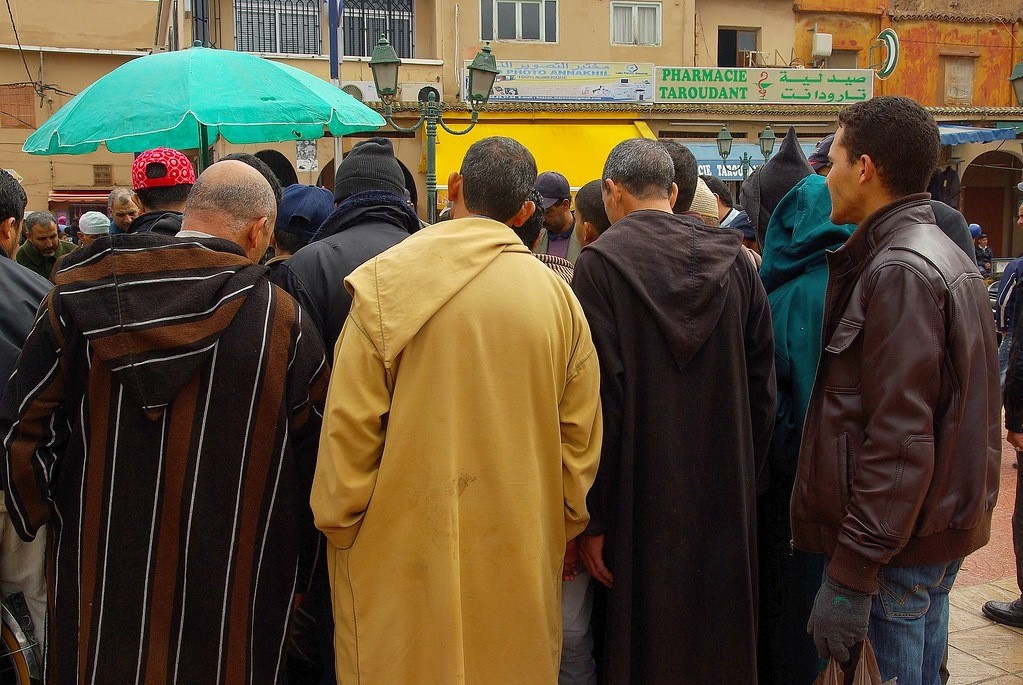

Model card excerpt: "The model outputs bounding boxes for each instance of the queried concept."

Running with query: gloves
[807,574,871,662]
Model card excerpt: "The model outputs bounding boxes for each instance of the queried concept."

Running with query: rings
[1015,447,1020,451]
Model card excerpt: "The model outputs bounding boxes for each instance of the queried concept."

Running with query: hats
[79,211,109,234]
[334,137,404,202]
[534,172,569,208]
[688,178,719,220]
[979,234,986,239]
[131,147,195,191]
[807,134,834,170]
[275,184,333,234]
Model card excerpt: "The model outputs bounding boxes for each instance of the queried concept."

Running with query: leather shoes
[981,598,1023,628]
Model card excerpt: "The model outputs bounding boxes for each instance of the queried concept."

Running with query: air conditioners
[399,82,444,103]
[338,79,382,103]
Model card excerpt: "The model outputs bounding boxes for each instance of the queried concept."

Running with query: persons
[982,267,1023,628]
[758,173,858,685]
[975,234,993,277]
[0,159,330,685]
[15,146,336,282]
[1017,202,1023,231]
[572,138,777,685]
[535,124,978,265]
[511,188,596,685]
[0,168,55,656]
[994,256,1023,375]
[273,137,420,685]
[309,137,603,685]
[790,94,1002,685]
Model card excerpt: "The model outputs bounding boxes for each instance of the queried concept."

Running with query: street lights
[366,35,502,223]
[711,123,778,181]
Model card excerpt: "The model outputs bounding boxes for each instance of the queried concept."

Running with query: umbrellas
[22,39,388,172]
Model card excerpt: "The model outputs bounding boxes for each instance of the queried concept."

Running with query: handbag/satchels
[813,636,882,685]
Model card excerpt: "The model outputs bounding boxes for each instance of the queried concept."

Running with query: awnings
[937,124,1020,202]
[687,143,817,181]
[420,119,658,210]
